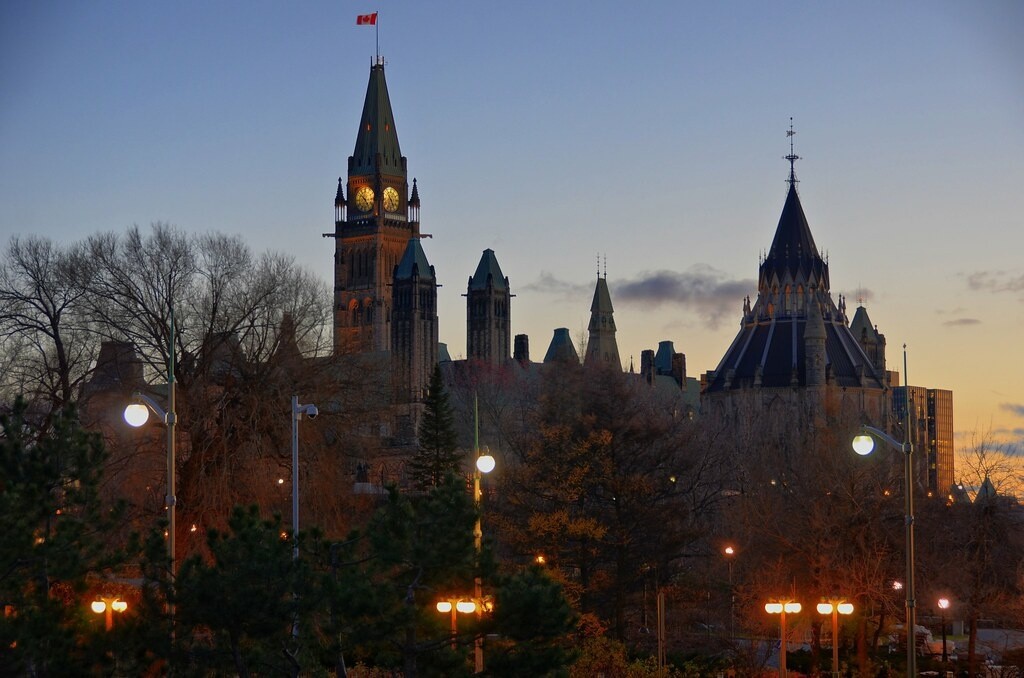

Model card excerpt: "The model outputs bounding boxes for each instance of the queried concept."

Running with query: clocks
[356,187,374,212]
[383,187,400,212]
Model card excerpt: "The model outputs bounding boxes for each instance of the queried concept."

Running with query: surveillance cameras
[307,407,318,419]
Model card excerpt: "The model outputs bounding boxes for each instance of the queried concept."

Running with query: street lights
[123,391,176,655]
[91,590,129,639]
[765,597,802,678]
[816,594,853,677]
[726,546,736,643]
[468,445,496,676]
[289,394,321,640]
[436,592,473,658]
[852,421,919,677]
[936,598,950,664]
